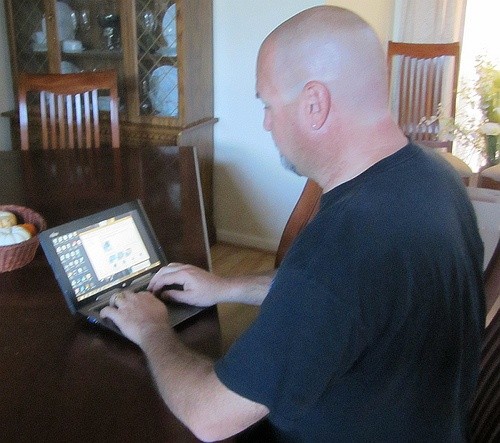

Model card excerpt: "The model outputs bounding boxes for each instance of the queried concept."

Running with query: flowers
[439,61,500,168]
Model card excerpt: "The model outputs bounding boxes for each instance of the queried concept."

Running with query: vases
[477,161,496,189]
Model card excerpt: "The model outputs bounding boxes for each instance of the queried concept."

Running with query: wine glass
[71,9,90,50]
[140,9,158,52]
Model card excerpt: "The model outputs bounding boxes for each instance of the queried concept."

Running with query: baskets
[0,204,46,273]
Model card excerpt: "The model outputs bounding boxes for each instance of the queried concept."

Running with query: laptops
[38,199,207,338]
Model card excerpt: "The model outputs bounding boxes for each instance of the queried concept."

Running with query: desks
[0,149,225,443]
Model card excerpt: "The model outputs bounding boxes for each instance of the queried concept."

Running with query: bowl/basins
[63,41,82,53]
[98,95,120,111]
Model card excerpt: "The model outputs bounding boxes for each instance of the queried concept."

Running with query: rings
[112,294,121,306]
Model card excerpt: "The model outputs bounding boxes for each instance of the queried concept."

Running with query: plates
[148,65,178,115]
[42,2,76,41]
[162,3,176,46]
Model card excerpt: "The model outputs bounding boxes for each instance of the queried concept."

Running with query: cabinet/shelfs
[6,0,218,247]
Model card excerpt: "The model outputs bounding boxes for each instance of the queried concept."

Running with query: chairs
[465,242,500,443]
[15,70,121,149]
[385,41,473,188]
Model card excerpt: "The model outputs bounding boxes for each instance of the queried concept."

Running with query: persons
[100,5,491,443]
